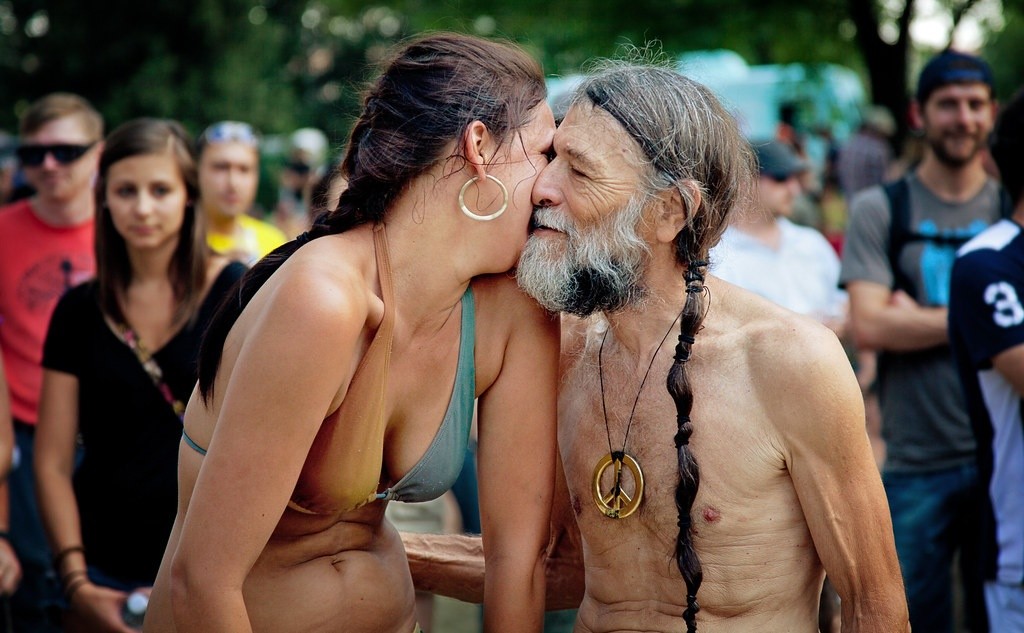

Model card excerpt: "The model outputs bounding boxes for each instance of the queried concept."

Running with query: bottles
[122,593,149,628]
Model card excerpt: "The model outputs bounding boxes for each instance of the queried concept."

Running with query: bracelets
[59,580,92,594]
[55,571,92,576]
[0,529,13,539]
[48,545,88,559]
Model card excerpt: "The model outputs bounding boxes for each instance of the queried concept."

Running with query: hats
[747,135,810,180]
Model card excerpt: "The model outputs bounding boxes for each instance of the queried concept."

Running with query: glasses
[196,121,261,151]
[16,140,99,166]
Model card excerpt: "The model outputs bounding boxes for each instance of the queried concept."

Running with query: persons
[524,62,918,633]
[275,123,337,235]
[0,93,105,600]
[190,114,287,267]
[710,138,857,361]
[141,28,563,633]
[35,119,259,633]
[840,49,1024,633]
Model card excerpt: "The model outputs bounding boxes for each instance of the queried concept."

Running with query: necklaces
[592,310,682,519]
